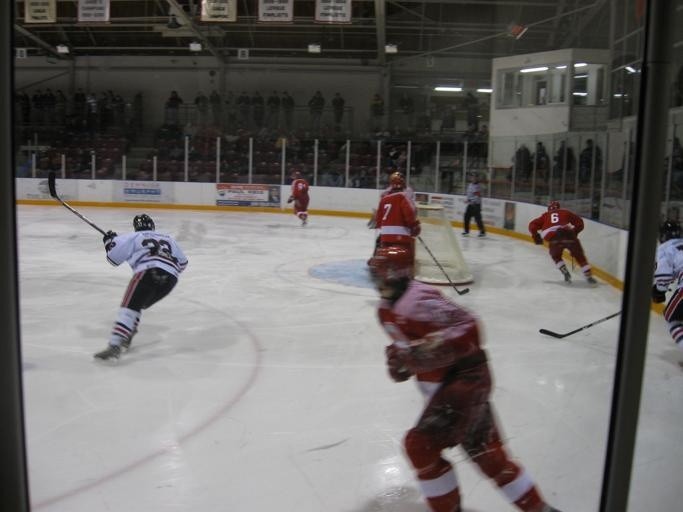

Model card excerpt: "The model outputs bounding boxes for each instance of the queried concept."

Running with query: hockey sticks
[571,254,577,271]
[48,170,108,236]
[418,235,469,295]
[539,311,622,339]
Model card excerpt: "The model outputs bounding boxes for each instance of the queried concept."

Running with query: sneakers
[587,278,596,284]
[94,345,120,361]
[462,233,468,235]
[122,331,136,348]
[565,272,568,282]
[478,235,484,237]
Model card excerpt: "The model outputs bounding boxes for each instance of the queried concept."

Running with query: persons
[461,172,488,238]
[366,247,563,511]
[651,221,683,368]
[95,213,189,361]
[285,170,311,228]
[508,138,604,186]
[529,201,598,285]
[611,138,682,203]
[12,90,481,197]
[368,171,423,281]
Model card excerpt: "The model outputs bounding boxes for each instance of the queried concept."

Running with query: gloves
[288,196,295,203]
[411,222,420,235]
[533,234,542,244]
[103,230,117,246]
[652,285,665,303]
[388,342,414,382]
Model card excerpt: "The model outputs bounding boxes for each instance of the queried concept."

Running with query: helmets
[548,201,560,210]
[390,172,405,190]
[659,221,679,243]
[133,213,155,231]
[291,171,301,179]
[367,247,409,285]
